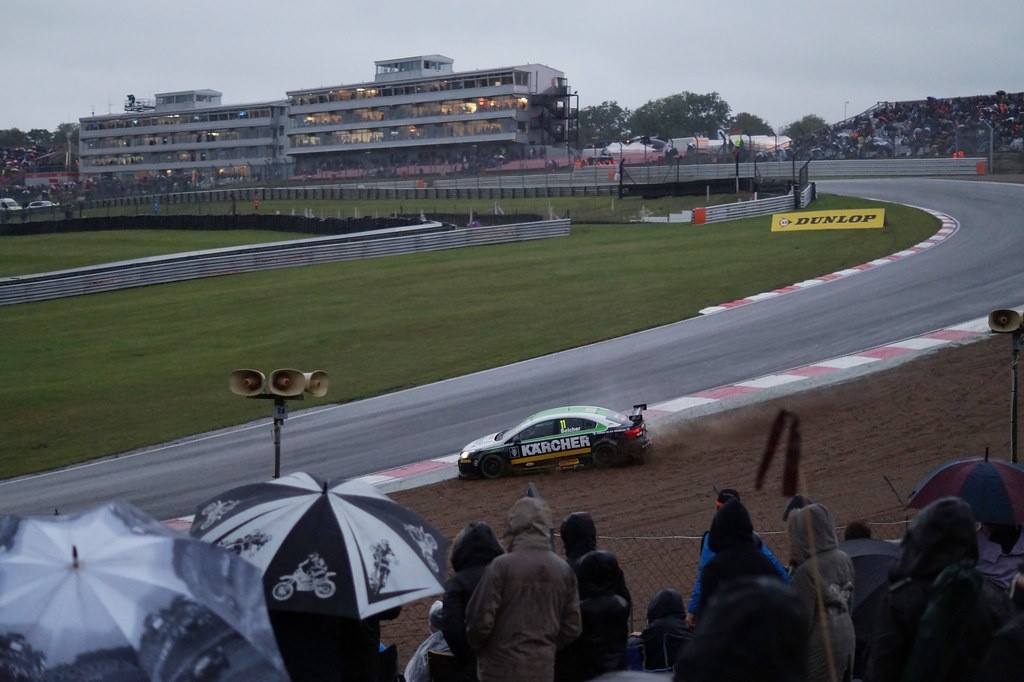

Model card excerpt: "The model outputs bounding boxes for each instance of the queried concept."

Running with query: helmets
[534,424,544,434]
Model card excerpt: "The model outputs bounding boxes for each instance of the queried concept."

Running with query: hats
[717,489,740,503]
[428,600,444,630]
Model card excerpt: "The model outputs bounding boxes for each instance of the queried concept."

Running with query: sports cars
[457,403,653,480]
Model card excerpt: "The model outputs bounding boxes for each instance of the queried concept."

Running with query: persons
[0,144,260,199]
[757,92,1023,162]
[291,95,547,178]
[402,499,632,682]
[627,489,1024,682]
[270,605,401,682]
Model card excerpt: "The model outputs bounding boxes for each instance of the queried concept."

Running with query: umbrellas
[996,90,1005,96]
[0,496,291,682]
[188,472,450,621]
[905,453,1024,525]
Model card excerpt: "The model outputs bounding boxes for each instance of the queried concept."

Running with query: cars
[25,201,58,210]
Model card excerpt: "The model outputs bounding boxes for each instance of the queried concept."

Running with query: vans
[0,198,23,211]
